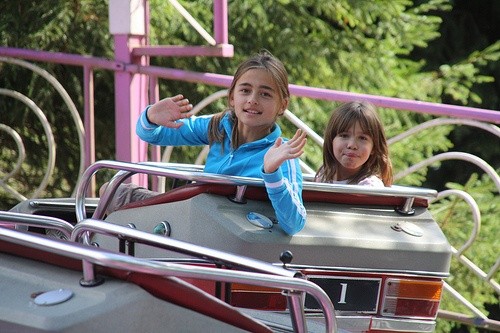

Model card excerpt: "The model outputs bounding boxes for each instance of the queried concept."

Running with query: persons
[313,99,396,188]
[136,51,308,237]
